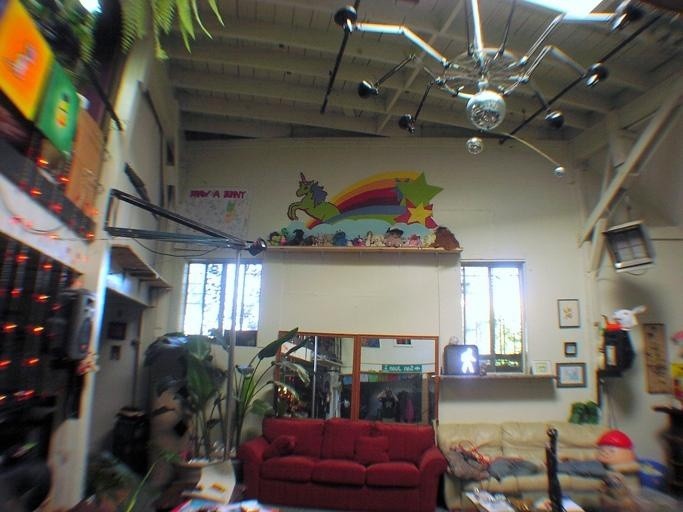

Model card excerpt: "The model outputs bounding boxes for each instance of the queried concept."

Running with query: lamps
[103,188,267,256]
[334,0,646,178]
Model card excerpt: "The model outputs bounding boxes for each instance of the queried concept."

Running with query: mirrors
[273,330,357,420]
[356,334,439,426]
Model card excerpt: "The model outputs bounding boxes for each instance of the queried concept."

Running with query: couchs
[436,422,641,511]
[237,416,447,512]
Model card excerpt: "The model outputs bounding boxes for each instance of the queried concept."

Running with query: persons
[375,385,397,420]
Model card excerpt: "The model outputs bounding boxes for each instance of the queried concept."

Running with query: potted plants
[142,325,313,483]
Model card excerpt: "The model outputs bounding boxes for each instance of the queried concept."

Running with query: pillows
[352,435,391,465]
[374,421,434,462]
[321,418,374,459]
[262,417,326,459]
[262,433,297,458]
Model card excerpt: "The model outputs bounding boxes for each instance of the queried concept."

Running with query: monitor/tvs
[444,345,480,374]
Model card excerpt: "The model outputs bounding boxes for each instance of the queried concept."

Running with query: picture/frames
[557,299,580,328]
[565,342,577,355]
[556,363,586,387]
